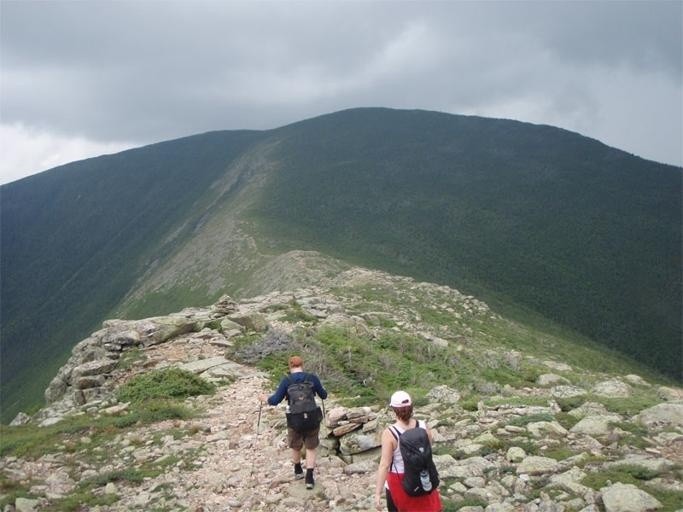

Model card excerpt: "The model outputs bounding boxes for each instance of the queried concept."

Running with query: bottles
[285,403,291,414]
[419,468,433,493]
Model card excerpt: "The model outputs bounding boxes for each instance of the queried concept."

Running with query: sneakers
[295,463,304,479]
[304,468,314,489]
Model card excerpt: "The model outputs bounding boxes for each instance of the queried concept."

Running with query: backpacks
[286,372,323,435]
[388,420,440,497]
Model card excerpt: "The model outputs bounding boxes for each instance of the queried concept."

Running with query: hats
[389,390,411,408]
[287,355,302,367]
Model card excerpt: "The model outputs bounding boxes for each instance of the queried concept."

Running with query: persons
[256,355,327,489]
[374,390,444,512]
